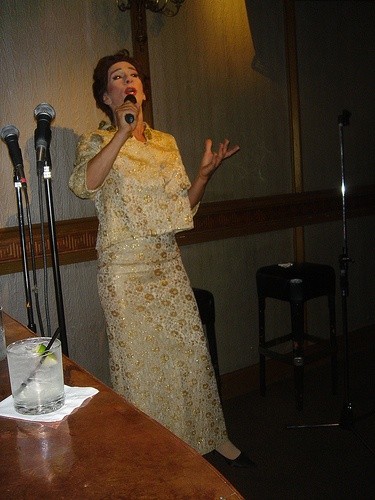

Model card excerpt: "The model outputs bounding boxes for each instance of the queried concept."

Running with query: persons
[68,50,257,471]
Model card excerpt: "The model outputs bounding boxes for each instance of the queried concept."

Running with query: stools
[190,287,220,393]
[256,262,336,408]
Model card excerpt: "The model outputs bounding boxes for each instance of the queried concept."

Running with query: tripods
[284,109,375,460]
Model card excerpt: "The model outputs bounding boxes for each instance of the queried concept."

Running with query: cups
[5,336,65,416]
[0,306,7,361]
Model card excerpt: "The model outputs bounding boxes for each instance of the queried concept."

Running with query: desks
[0,311,242,500]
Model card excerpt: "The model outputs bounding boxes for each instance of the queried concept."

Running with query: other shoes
[210,448,255,468]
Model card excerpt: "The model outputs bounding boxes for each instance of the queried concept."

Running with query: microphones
[123,94,137,124]
[33,102,56,164]
[0,124,27,187]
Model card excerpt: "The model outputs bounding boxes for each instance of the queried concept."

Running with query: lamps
[116,0,185,51]
